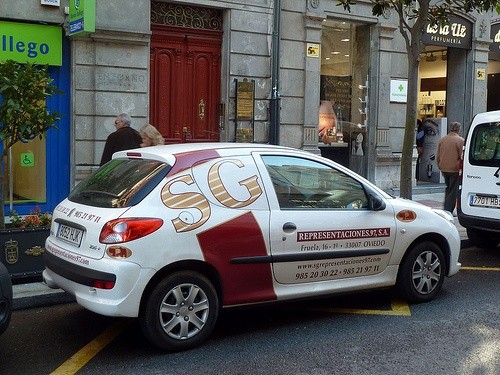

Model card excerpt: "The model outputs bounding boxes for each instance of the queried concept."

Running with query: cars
[44,140,465,350]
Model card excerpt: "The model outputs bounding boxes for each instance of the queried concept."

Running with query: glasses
[114,120,122,125]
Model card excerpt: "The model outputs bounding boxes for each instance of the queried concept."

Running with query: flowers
[0,58,65,228]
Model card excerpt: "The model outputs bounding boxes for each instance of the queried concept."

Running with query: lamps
[442,50,447,60]
[426,52,436,61]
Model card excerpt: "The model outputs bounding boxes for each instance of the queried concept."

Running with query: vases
[0,224,52,280]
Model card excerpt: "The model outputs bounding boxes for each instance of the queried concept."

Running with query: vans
[458,109,500,244]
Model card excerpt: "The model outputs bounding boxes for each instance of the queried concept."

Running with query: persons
[100,113,143,167]
[140,124,165,147]
[418,117,447,183]
[416,119,426,181]
[436,122,465,217]
